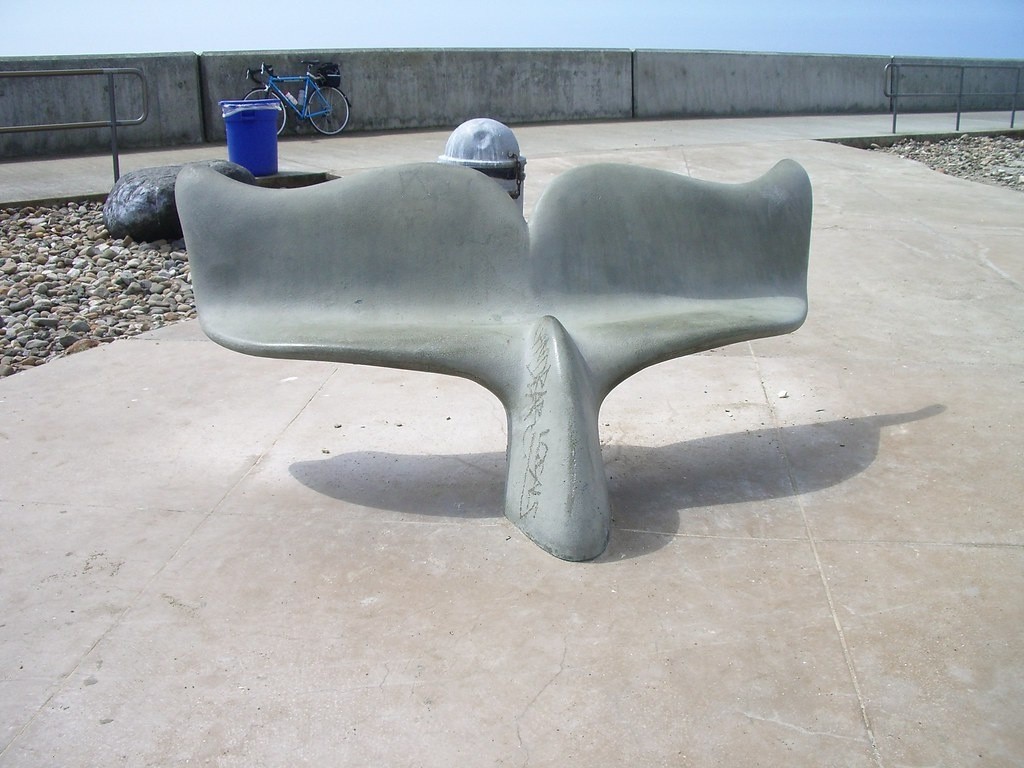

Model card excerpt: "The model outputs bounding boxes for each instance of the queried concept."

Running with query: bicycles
[243,58,353,138]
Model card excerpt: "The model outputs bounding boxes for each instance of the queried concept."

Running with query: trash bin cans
[219,99,278,178]
